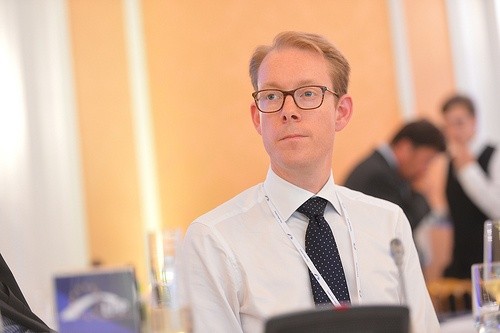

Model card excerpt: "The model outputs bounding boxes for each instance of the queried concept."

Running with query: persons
[440,95,500,280]
[343,120,447,283]
[175,31,443,333]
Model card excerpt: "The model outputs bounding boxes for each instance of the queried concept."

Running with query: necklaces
[261,187,365,307]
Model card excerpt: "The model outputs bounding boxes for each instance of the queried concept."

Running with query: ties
[295,197,353,311]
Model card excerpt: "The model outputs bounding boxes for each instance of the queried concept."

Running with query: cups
[483,220,500,299]
[471,262,500,333]
[144,224,180,309]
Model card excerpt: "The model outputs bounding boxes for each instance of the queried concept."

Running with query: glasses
[251,85,341,113]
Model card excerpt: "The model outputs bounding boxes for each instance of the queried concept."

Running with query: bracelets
[451,156,462,164]
